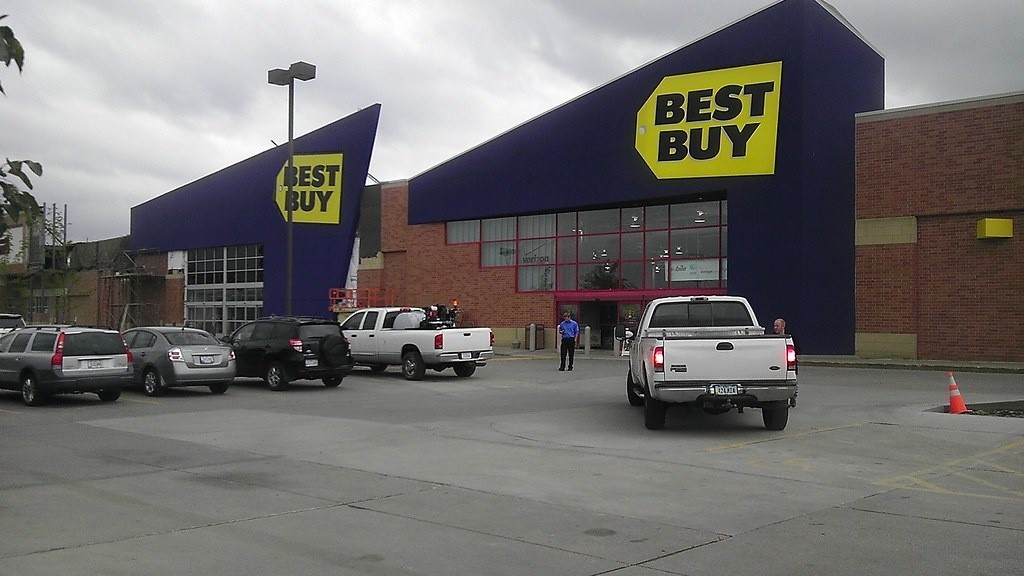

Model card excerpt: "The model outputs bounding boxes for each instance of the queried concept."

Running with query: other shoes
[559,367,571,371]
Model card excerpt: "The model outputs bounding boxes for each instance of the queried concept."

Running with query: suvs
[0,313,27,337]
[221,314,355,392]
[0,323,135,406]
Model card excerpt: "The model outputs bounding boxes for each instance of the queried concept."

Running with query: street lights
[268,61,316,316]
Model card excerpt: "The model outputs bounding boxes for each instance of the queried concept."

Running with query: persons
[773,319,800,407]
[559,311,579,371]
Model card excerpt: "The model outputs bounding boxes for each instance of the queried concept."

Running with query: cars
[118,326,237,397]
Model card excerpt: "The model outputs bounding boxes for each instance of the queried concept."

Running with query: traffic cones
[947,372,973,415]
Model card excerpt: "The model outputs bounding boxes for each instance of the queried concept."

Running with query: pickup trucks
[614,294,797,432]
[340,307,494,381]
